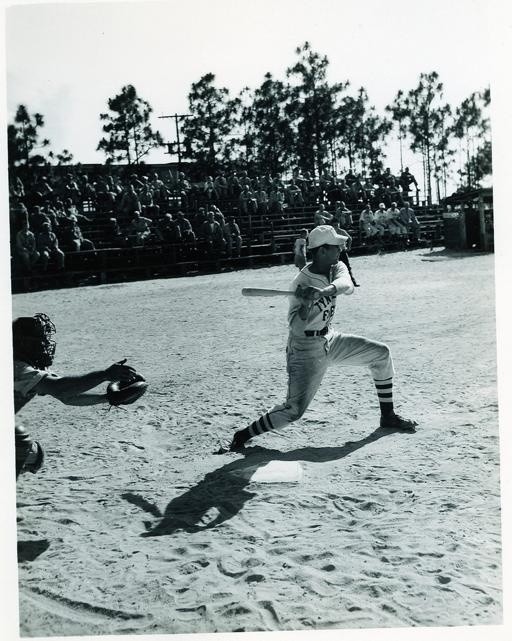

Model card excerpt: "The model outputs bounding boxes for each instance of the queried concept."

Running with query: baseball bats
[242,289,319,299]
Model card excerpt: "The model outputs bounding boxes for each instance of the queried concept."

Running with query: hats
[305,225,348,250]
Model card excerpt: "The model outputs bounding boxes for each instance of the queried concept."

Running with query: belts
[305,326,328,336]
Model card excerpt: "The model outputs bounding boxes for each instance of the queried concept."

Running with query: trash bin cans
[443,207,486,253]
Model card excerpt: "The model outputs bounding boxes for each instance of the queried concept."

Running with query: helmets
[14,317,56,361]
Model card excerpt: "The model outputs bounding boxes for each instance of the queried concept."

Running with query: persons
[234,226,418,449]
[293,228,308,271]
[239,167,421,239]
[9,169,243,275]
[329,218,360,287]
[13,313,136,475]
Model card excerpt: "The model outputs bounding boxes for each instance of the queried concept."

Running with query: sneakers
[231,432,245,450]
[380,411,415,429]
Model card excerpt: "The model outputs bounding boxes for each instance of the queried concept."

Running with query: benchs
[9,165,447,293]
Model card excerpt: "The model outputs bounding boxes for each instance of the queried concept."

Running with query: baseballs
[473,244,476,247]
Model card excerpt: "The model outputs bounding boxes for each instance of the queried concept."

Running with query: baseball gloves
[107,372,148,410]
